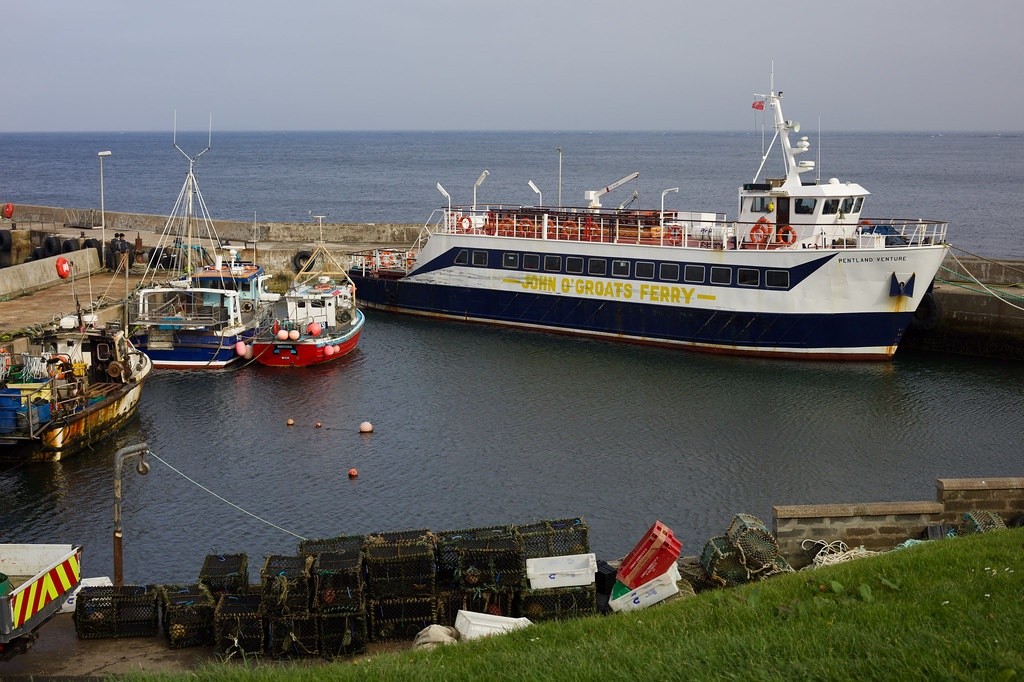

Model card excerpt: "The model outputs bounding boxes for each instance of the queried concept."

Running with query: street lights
[97,150,112,270]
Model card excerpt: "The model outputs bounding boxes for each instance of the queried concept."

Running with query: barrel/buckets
[0,386,51,436]
[212,307,228,330]
[197,306,213,330]
[0,572,14,598]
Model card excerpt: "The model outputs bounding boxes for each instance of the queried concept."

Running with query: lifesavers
[0,348,10,372]
[538,219,555,237]
[584,222,599,239]
[667,224,682,244]
[757,218,772,234]
[562,221,577,238]
[459,215,472,232]
[500,218,514,234]
[519,218,533,236]
[860,220,872,224]
[749,225,768,243]
[778,226,797,246]
[381,252,393,266]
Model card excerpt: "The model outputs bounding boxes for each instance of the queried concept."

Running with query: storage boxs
[73,514,699,658]
[1,389,51,436]
[72,363,88,377]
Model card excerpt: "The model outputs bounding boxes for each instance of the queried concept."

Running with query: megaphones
[785,121,800,133]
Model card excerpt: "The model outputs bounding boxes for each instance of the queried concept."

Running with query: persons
[794,198,814,215]
[118,233,132,273]
[108,232,122,274]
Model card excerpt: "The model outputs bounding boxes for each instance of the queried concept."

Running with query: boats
[335,56,953,364]
[248,211,366,371]
[127,107,275,372]
[0,239,151,454]
